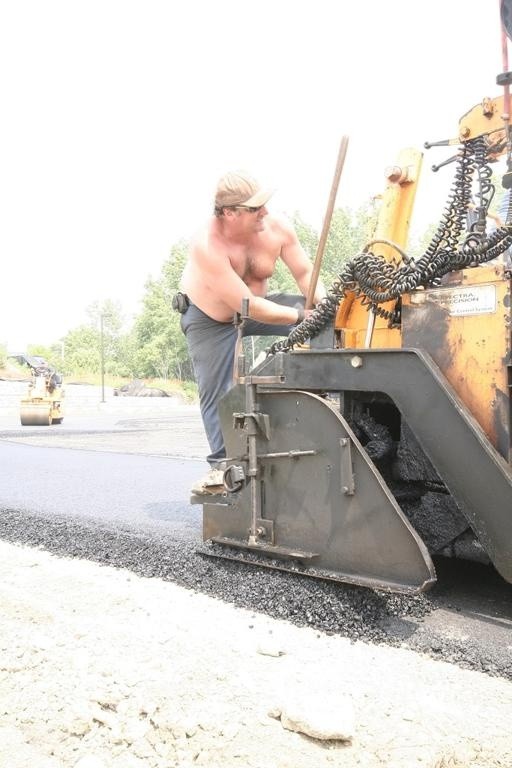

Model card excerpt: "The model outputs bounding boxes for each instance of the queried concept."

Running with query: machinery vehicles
[7,353,64,425]
[190,0,512,599]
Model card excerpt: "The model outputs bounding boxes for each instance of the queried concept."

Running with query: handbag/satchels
[172,295,188,313]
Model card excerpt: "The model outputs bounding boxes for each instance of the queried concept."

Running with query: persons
[172,172,325,472]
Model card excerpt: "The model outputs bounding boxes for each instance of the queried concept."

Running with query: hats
[216,173,271,208]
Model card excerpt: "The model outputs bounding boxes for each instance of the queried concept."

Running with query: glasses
[235,208,258,212]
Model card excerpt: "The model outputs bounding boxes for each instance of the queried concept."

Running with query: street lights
[101,313,112,402]
